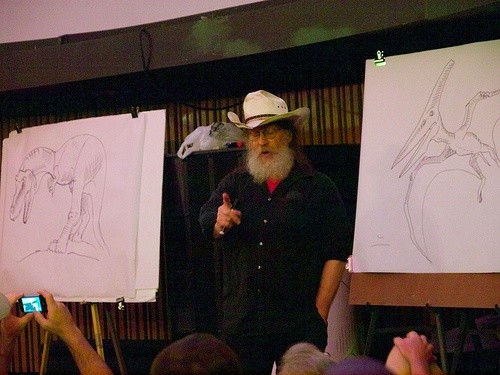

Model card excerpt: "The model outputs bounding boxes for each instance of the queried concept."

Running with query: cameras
[16,295,48,314]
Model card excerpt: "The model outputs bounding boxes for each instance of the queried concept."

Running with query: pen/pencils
[220,197,240,234]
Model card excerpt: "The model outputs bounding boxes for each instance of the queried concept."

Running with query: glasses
[244,124,281,142]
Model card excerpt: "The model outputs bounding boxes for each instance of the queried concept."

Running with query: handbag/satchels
[177,122,241,159]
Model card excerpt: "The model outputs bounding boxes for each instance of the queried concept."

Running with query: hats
[227,89,310,129]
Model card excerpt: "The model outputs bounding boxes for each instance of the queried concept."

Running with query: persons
[150,330,445,375]
[198,90,353,375]
[0,290,115,375]
